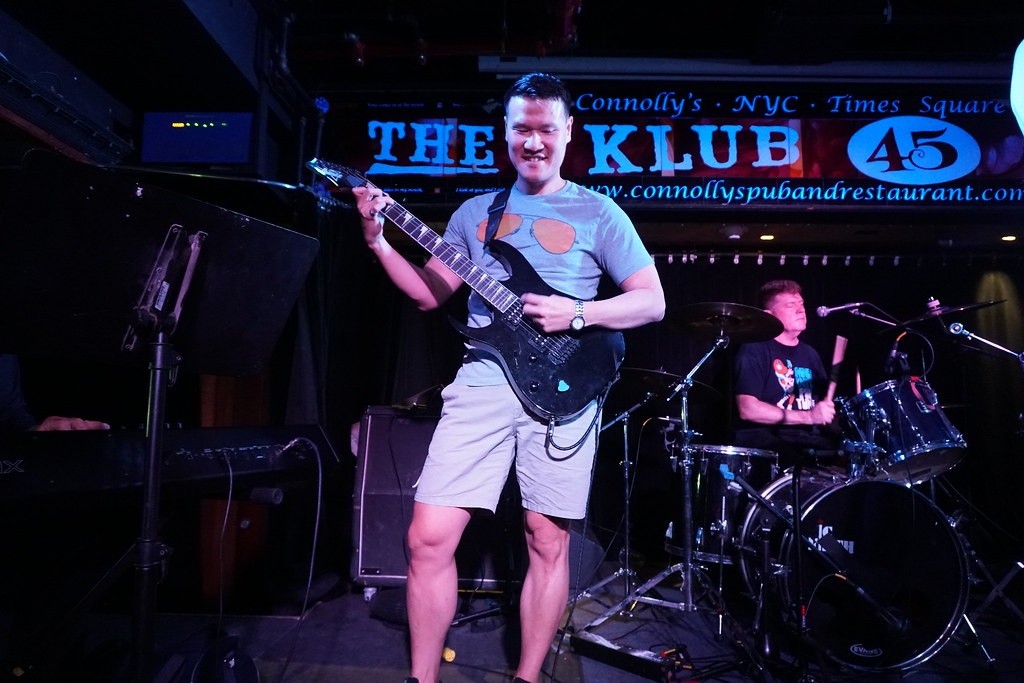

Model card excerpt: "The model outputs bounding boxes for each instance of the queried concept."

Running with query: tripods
[564,328,778,683]
[1,173,325,683]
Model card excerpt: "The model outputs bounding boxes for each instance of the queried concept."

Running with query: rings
[369,210,378,217]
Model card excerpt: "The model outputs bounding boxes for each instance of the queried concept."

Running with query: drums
[663,442,781,567]
[736,469,973,675]
[836,375,971,489]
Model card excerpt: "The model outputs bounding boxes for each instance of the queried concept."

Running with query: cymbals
[677,299,785,345]
[875,295,1008,337]
[618,365,720,403]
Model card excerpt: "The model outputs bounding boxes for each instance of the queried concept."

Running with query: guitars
[303,155,628,429]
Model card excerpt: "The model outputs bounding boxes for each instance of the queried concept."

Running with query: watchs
[571,300,585,331]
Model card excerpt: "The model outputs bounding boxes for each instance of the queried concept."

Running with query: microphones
[884,340,899,373]
[263,438,299,464]
[860,592,913,641]
[817,301,864,317]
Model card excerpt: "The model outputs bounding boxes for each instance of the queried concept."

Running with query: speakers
[349,406,530,592]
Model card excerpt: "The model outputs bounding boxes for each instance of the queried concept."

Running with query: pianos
[0,422,339,505]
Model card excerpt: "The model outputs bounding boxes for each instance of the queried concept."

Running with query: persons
[350,73,666,683]
[729,279,839,467]
